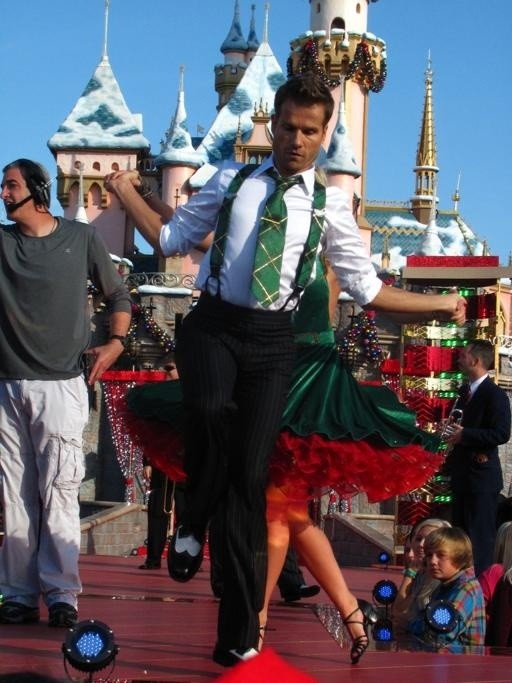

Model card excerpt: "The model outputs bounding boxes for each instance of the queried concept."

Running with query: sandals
[343,598,379,665]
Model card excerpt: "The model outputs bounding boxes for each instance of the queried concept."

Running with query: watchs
[111,334,130,353]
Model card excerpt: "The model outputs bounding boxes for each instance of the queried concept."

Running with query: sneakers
[47,600,80,629]
[0,598,41,625]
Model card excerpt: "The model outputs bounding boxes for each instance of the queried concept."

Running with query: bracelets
[141,185,154,200]
[404,568,417,579]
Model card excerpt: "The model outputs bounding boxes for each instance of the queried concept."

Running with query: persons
[395,525,487,644]
[485,523,511,646]
[392,517,452,625]
[0,157,138,632]
[100,74,470,666]
[476,520,512,609]
[438,338,511,577]
[277,537,321,603]
[107,160,472,664]
[133,349,181,571]
[387,531,415,617]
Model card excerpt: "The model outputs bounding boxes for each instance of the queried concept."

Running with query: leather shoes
[284,583,320,602]
[168,521,206,582]
[213,640,260,668]
[139,560,162,569]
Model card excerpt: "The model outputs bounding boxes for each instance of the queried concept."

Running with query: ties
[250,166,304,309]
